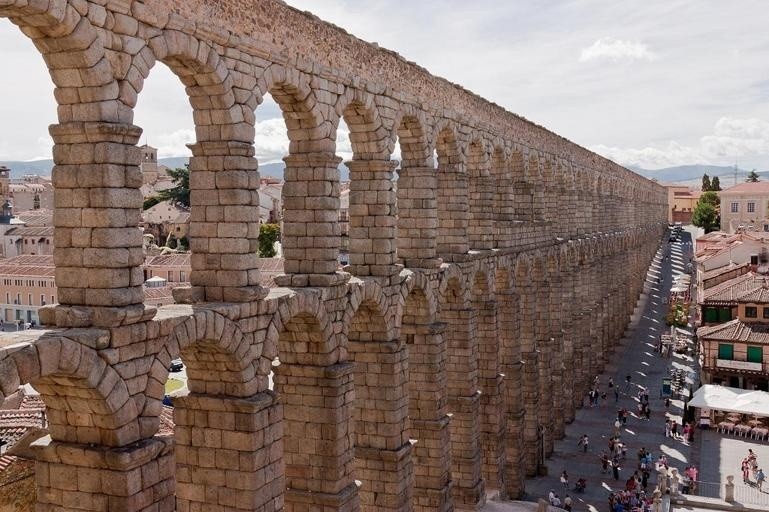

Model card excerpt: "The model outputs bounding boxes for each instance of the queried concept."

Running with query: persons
[660,275,664,283]
[548,373,699,512]
[31,320,34,328]
[665,256,669,263]
[660,255,664,263]
[0,320,4,331]
[741,448,768,492]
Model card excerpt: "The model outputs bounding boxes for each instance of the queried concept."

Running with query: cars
[163,394,173,406]
[168,361,183,372]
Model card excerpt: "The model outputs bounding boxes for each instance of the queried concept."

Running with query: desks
[713,411,768,442]
